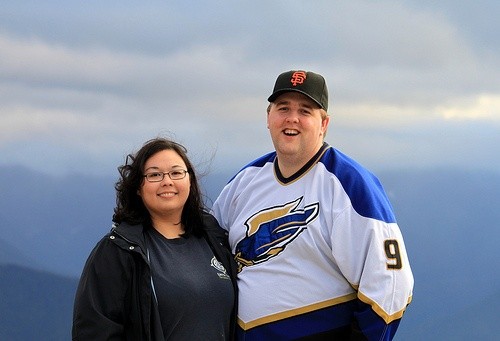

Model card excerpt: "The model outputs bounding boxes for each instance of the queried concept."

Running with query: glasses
[141,169,190,182]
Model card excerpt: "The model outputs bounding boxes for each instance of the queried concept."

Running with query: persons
[207,70,415,341]
[72,137,239,341]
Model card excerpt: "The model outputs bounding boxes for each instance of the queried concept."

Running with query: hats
[268,70,329,112]
